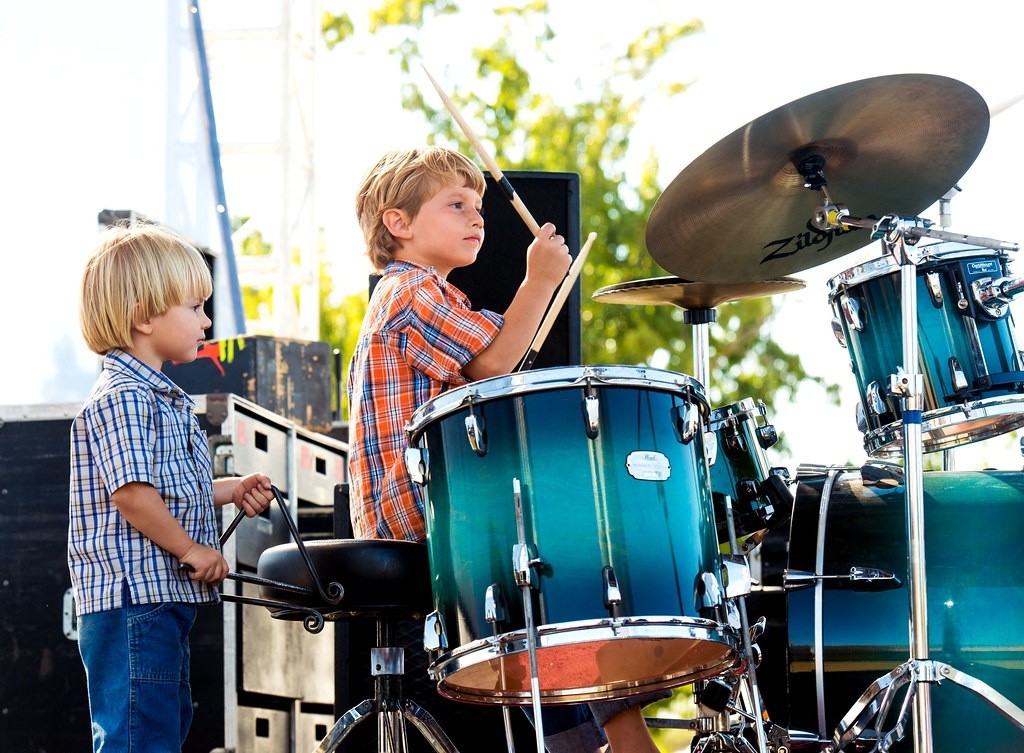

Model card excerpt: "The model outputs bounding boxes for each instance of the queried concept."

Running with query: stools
[257,538,456,753]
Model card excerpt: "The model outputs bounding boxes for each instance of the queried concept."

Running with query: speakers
[445,171,581,369]
[0,404,349,753]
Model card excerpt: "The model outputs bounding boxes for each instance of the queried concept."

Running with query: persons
[68,222,275,753]
[346,144,672,753]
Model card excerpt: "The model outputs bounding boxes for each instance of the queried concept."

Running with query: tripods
[813,184,1024,753]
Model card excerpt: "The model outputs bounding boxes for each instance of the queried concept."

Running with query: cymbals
[643,72,992,282]
[592,272,812,308]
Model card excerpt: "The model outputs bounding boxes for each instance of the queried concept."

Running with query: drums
[702,395,778,547]
[827,240,1024,455]
[402,362,747,707]
[781,463,1023,752]
